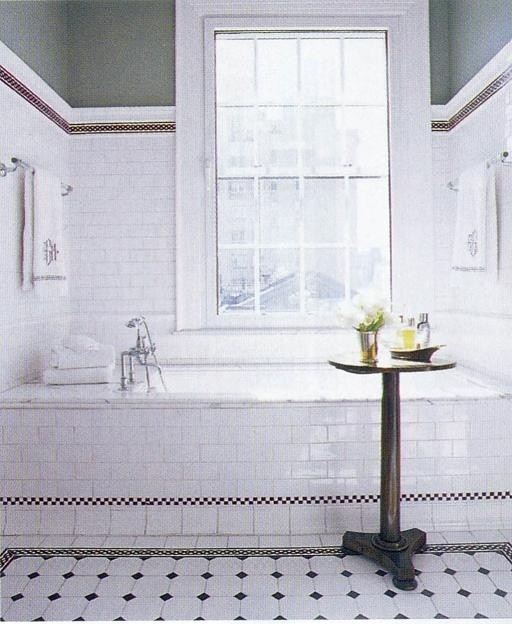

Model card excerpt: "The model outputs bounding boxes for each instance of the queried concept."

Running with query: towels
[22,165,67,295]
[41,332,115,385]
[450,157,499,277]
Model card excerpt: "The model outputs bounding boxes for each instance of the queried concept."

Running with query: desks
[328,353,458,591]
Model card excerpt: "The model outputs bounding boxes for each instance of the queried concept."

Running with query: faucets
[118,343,163,390]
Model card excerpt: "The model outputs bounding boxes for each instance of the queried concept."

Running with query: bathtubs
[135,358,506,408]
